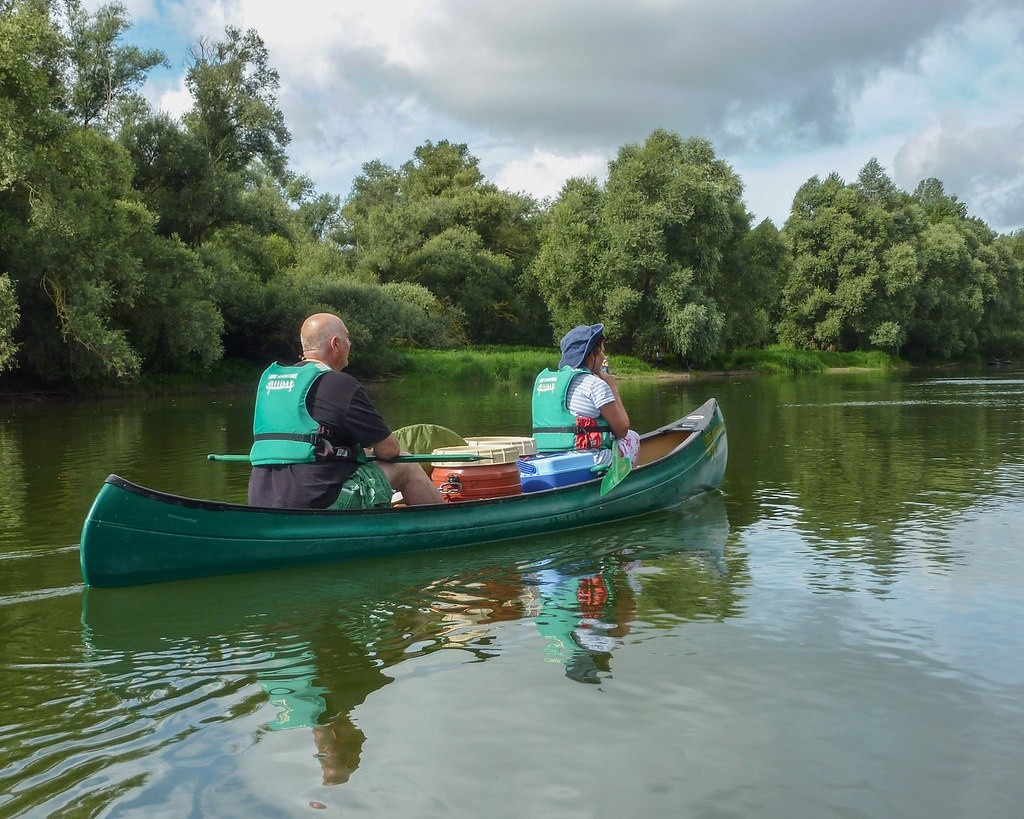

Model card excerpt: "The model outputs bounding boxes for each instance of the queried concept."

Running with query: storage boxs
[518,452,598,494]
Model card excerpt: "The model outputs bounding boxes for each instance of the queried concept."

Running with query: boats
[81,397,728,588]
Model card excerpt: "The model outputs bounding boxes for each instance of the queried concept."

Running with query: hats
[558,324,605,371]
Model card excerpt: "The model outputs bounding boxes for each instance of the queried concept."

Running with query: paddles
[206,451,500,462]
[598,437,633,497]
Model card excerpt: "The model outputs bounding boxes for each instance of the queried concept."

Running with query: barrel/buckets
[430,433,539,502]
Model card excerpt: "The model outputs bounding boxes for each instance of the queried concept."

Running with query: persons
[254,595,446,789]
[530,319,639,476]
[245,310,450,507]
[535,541,637,685]
